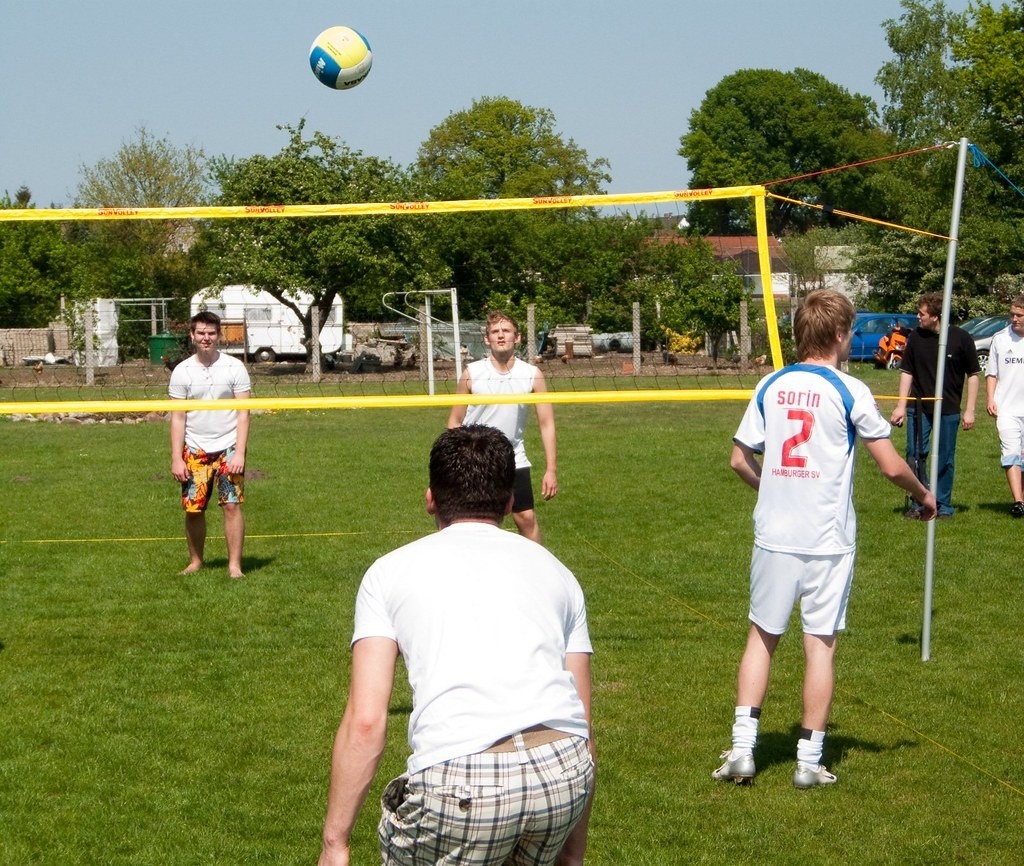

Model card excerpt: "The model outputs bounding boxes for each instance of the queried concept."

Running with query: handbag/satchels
[909,457,924,483]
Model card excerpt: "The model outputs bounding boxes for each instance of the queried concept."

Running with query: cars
[591,331,633,351]
[844,313,918,362]
[958,315,1012,372]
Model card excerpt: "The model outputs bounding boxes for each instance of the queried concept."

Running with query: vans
[190,285,344,364]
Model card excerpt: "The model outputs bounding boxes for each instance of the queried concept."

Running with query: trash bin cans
[148,333,189,365]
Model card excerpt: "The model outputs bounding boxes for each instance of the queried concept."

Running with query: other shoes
[1010,501,1024,518]
[906,509,920,519]
[939,515,951,520]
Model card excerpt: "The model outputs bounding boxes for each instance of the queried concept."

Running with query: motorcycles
[872,316,912,371]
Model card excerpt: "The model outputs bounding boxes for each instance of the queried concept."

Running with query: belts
[483,724,575,753]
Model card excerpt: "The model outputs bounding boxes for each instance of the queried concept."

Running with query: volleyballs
[308,25,375,92]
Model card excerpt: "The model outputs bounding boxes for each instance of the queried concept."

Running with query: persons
[889,293,982,520]
[316,424,594,866]
[169,311,250,579]
[985,296,1024,519]
[713,289,937,790]
[447,312,559,545]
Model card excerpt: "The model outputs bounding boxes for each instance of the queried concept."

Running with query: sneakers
[712,750,756,784]
[792,759,837,788]
